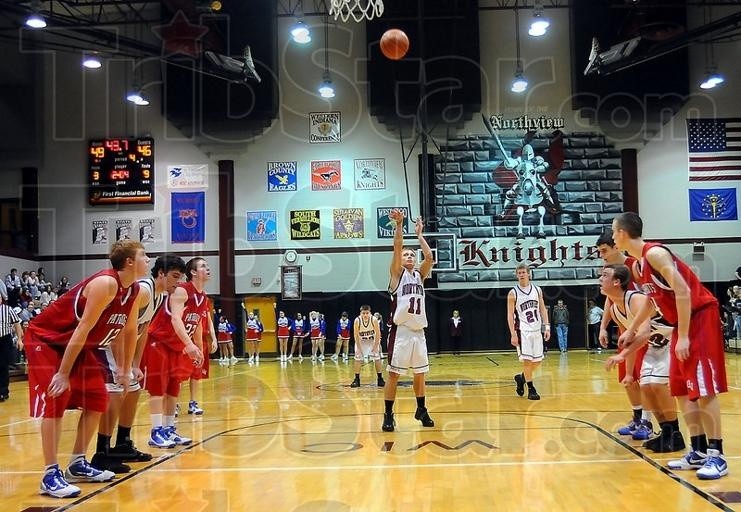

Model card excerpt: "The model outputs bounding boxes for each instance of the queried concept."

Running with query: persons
[598,263,687,454]
[91,252,187,477]
[721,282,741,352]
[611,212,730,481]
[553,299,571,354]
[0,264,73,403]
[597,233,660,442]
[448,308,467,358]
[173,297,218,420]
[25,239,151,498]
[133,255,212,450]
[382,208,438,432]
[350,304,387,388]
[208,299,392,365]
[506,264,551,401]
[586,299,606,350]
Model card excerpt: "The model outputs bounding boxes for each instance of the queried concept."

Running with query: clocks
[284,250,298,265]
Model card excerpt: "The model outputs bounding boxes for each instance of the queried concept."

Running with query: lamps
[511,9,527,93]
[26,14,47,28]
[700,5,724,89]
[126,23,149,105]
[82,55,101,69]
[290,0,311,44]
[319,15,335,98]
[528,0,550,37]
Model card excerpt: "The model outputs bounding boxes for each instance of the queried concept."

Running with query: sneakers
[414,407,434,428]
[632,419,653,439]
[65,455,115,482]
[114,440,152,461]
[90,451,131,473]
[514,373,525,396]
[617,418,639,435]
[695,449,728,478]
[668,445,706,470]
[643,427,685,453]
[378,378,386,387]
[351,378,360,387]
[528,387,540,400]
[39,464,81,499]
[164,425,193,444]
[148,427,176,448]
[382,411,396,431]
[218,353,385,365]
[175,403,181,418]
[189,401,203,415]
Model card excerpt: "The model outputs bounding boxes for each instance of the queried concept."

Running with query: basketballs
[380,29,410,61]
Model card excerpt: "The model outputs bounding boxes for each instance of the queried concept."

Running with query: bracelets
[546,324,550,331]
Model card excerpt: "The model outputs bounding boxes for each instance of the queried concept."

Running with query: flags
[685,117,741,183]
[689,187,739,222]
